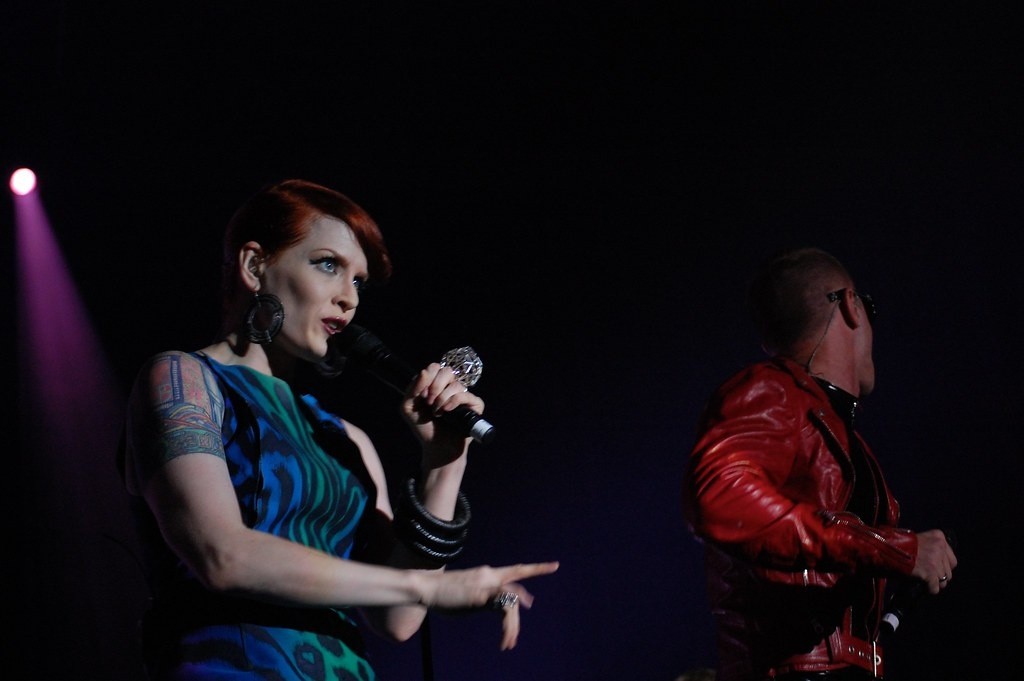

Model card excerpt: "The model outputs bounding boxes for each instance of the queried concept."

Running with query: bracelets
[409,476,473,558]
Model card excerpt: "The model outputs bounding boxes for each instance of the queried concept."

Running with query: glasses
[829,289,880,319]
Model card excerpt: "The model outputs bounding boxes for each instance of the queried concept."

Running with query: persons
[681,247,957,681]
[123,181,559,681]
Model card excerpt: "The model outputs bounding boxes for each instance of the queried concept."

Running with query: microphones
[336,324,495,445]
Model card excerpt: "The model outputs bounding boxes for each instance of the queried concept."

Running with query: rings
[939,576,947,582]
[493,591,514,611]
